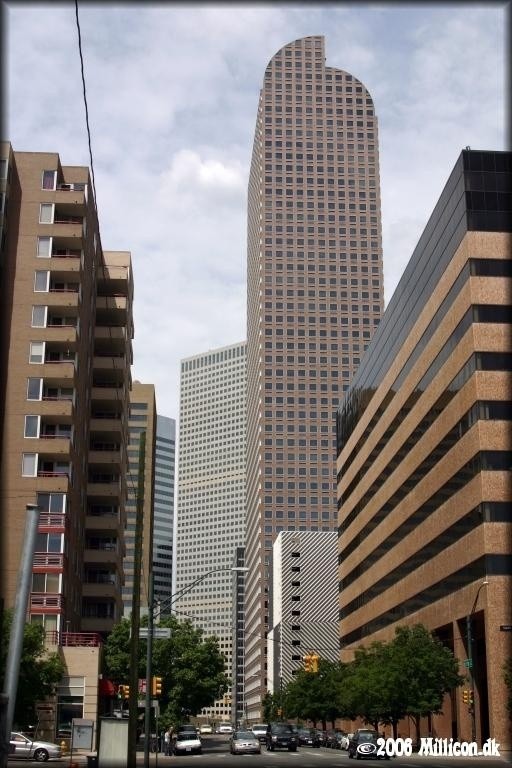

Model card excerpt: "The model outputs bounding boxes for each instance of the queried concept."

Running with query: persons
[164,728,170,756]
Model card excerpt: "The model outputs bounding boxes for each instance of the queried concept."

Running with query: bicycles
[151,739,160,753]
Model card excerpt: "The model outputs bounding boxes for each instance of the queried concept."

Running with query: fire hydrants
[61,740,68,756]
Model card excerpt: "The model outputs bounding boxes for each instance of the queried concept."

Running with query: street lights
[143,566,252,766]
[465,579,490,752]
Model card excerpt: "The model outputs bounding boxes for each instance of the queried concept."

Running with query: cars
[173,720,392,761]
[8,730,61,762]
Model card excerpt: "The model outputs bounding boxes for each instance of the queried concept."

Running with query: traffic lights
[462,682,474,709]
[151,674,164,696]
[118,684,131,701]
[300,654,321,673]
